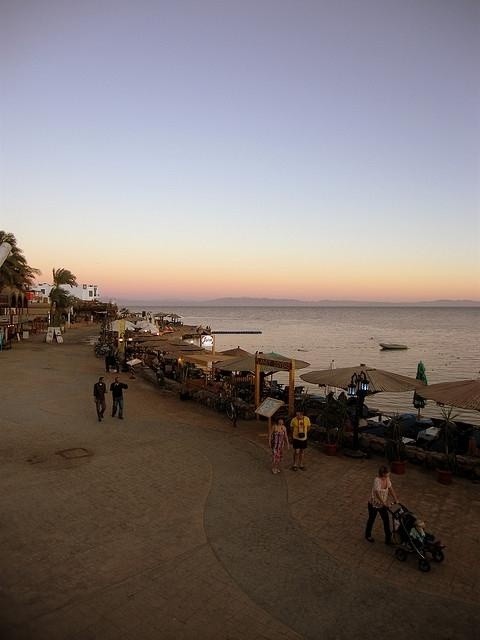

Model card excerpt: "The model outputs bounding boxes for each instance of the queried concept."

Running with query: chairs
[267,379,305,411]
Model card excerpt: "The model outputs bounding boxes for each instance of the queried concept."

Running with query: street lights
[118,332,133,371]
[347,371,369,459]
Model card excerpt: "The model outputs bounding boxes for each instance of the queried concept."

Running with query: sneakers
[366,536,396,546]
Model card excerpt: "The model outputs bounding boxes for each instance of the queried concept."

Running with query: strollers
[387,501,446,572]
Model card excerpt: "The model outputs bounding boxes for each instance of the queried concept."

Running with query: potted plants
[436,406,459,485]
[315,398,351,456]
[384,407,407,475]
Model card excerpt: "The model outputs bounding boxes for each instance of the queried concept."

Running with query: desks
[416,426,441,441]
[402,436,416,445]
[367,415,391,423]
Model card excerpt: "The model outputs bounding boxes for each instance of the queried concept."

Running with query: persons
[409,518,427,545]
[289,407,312,472]
[269,415,290,476]
[110,375,128,421]
[364,465,399,546]
[93,376,108,422]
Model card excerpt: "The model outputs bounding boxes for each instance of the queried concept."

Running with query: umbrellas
[298,363,426,397]
[412,362,429,419]
[325,361,339,396]
[414,379,480,410]
[153,311,184,323]
[103,314,168,341]
[134,336,311,373]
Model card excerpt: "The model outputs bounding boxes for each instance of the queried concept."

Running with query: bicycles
[226,397,237,427]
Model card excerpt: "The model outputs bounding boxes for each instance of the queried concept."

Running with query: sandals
[271,466,307,474]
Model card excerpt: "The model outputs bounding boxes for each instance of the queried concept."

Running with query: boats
[379,343,408,350]
